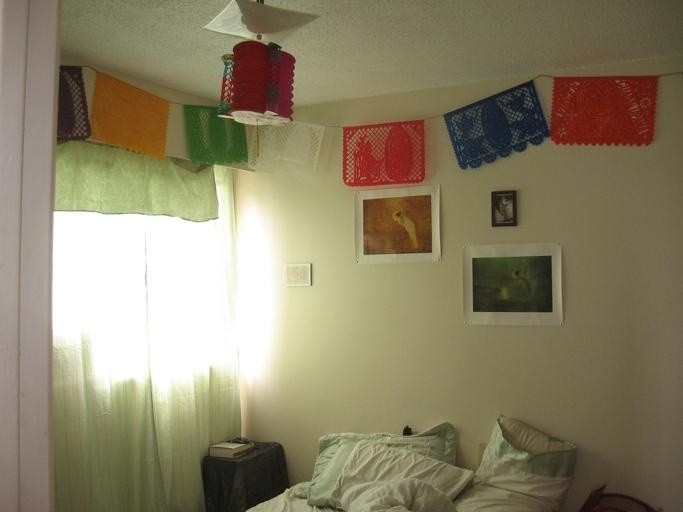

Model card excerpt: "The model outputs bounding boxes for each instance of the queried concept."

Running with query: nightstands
[202,439,290,512]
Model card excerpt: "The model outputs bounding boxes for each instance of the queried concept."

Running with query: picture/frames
[490,187,519,228]
[286,261,314,288]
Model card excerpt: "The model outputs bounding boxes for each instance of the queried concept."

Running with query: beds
[243,479,336,511]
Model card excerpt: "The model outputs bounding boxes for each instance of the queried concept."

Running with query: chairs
[579,483,657,512]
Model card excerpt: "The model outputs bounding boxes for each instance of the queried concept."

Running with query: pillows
[309,441,474,511]
[475,413,578,511]
[309,421,459,498]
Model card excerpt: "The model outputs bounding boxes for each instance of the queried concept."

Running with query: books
[209,442,254,459]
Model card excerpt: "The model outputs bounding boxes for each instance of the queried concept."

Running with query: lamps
[203,0,298,129]
